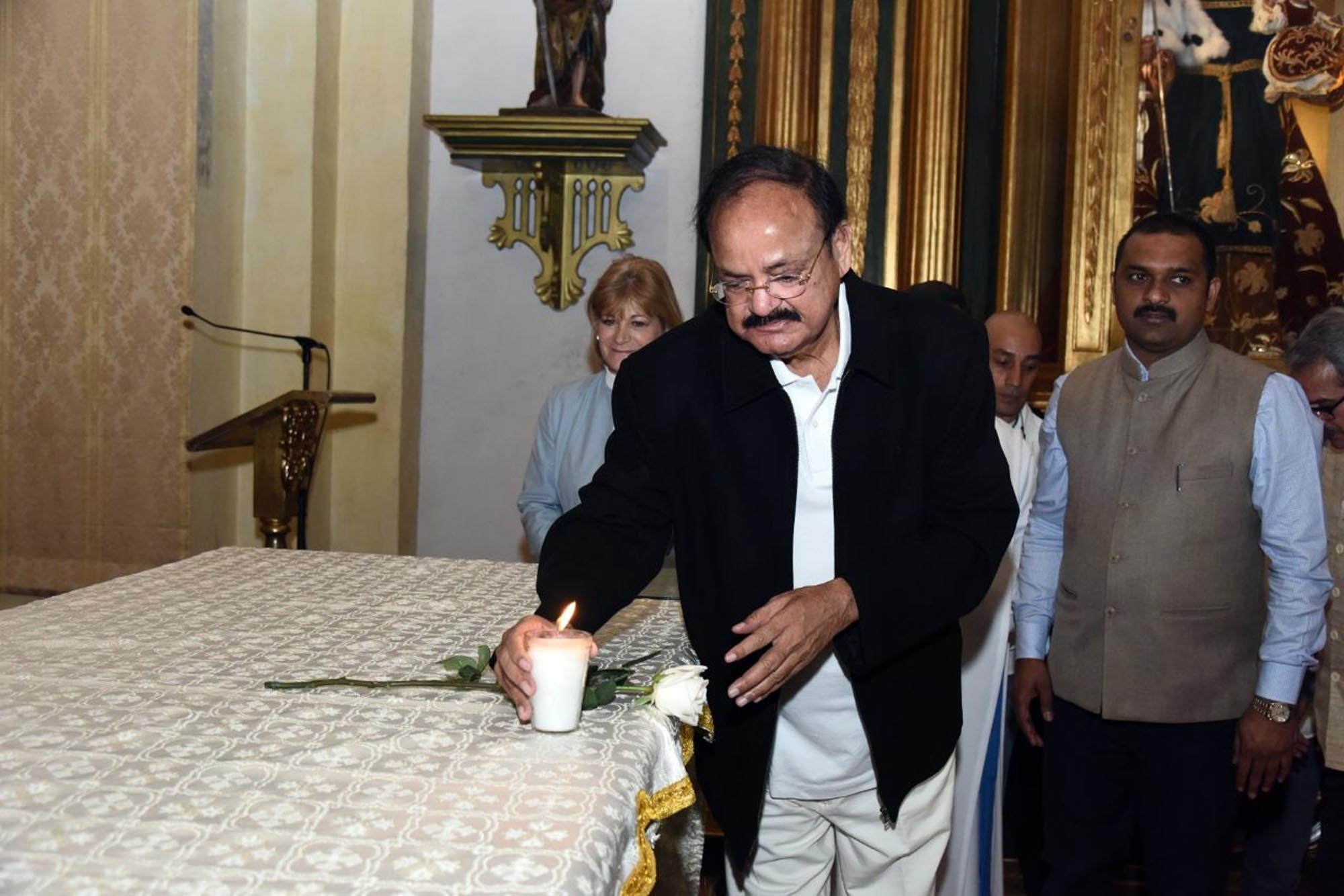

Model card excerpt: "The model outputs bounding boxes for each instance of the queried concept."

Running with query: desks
[2,548,717,896]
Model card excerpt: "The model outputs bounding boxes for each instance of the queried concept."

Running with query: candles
[529,623,590,730]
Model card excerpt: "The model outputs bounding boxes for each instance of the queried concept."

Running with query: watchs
[1252,696,1294,723]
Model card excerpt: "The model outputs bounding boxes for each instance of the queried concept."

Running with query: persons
[515,256,683,564]
[907,282,1344,896]
[1016,212,1334,896]
[492,148,1020,896]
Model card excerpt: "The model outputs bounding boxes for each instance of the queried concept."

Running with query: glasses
[1309,398,1344,421]
[706,232,830,308]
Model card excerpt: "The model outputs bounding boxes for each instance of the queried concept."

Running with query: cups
[525,630,593,733]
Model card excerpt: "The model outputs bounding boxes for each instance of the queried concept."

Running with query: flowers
[264,643,708,726]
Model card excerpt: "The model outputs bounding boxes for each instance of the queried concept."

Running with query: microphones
[179,305,326,348]
